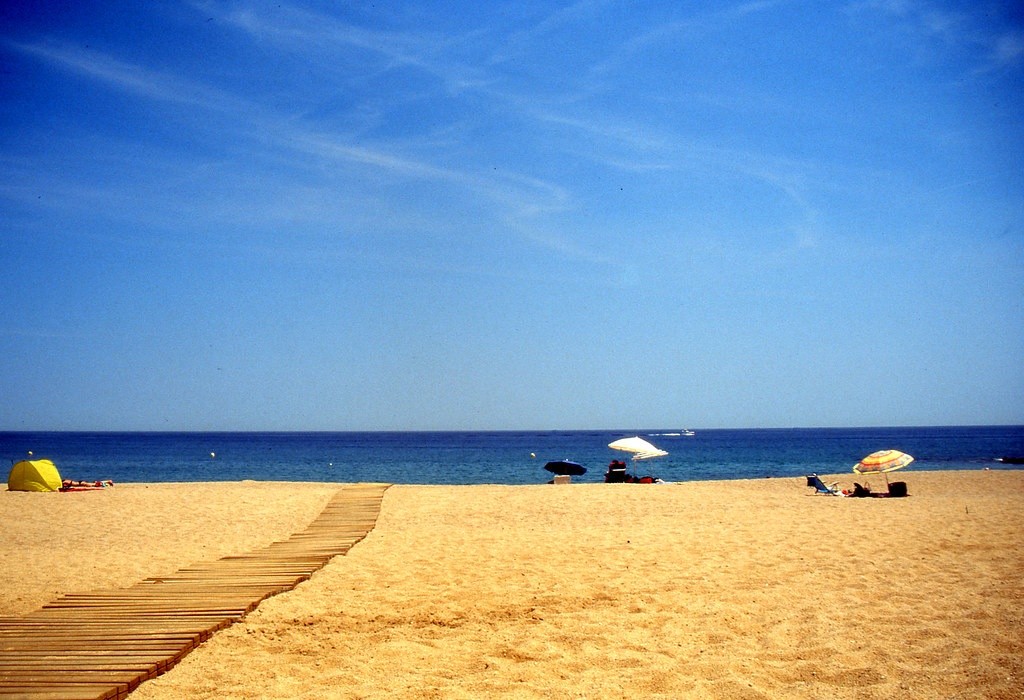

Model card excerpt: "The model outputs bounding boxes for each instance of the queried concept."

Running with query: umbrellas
[544,459,587,476]
[854,450,914,491]
[608,436,669,476]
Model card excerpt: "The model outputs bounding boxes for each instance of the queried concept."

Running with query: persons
[63,479,113,487]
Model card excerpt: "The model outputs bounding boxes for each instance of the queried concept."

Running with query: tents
[8,458,62,492]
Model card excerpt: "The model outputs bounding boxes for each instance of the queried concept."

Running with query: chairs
[605,461,626,483]
[806,473,839,496]
